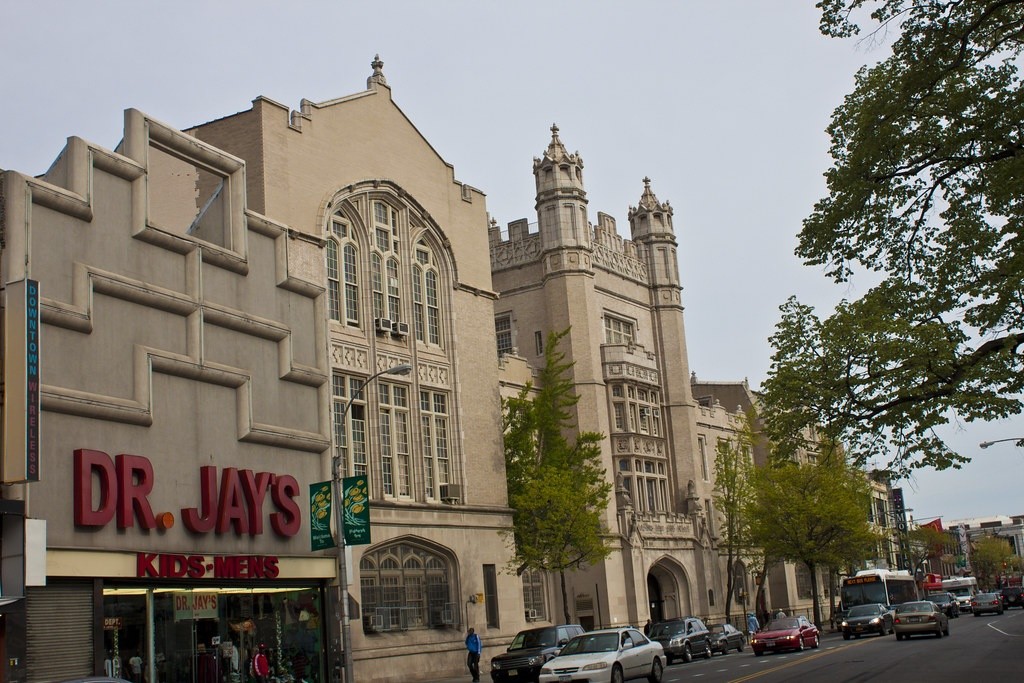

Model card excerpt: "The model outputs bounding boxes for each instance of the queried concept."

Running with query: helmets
[259,642,268,649]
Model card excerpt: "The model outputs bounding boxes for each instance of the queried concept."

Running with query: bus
[840,569,919,618]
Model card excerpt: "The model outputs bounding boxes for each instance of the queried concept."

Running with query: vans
[942,576,979,613]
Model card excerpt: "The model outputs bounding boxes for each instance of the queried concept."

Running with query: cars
[842,603,894,641]
[706,624,746,655]
[972,593,1003,617]
[539,627,666,683]
[893,600,949,641]
[751,616,820,656]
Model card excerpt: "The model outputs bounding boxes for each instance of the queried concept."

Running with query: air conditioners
[375,318,409,337]
[441,610,452,624]
[370,615,384,632]
[525,609,536,618]
[441,484,461,500]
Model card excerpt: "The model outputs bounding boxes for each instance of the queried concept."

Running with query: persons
[747,608,786,642]
[465,628,482,683]
[644,619,653,636]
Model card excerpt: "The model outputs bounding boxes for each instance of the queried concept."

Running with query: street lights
[334,363,415,683]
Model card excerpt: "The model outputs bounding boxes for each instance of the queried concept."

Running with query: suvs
[922,592,959,619]
[490,625,586,683]
[1002,586,1024,610]
[648,617,712,664]
